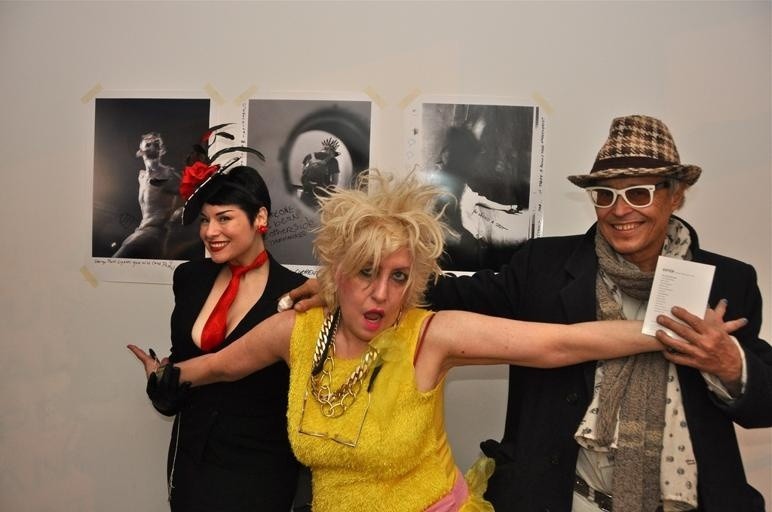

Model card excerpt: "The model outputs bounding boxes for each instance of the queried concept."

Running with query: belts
[575,478,615,512]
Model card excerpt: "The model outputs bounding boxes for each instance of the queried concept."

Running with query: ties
[201,249,269,352]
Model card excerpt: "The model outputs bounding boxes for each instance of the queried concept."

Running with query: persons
[128,169,749,511]
[164,166,314,510]
[278,115,772,511]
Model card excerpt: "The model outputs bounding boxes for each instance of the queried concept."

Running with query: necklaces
[306,303,402,417]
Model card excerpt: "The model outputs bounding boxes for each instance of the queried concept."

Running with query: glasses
[298,382,371,448]
[583,181,670,207]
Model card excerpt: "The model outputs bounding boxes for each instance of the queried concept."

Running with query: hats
[569,115,702,189]
